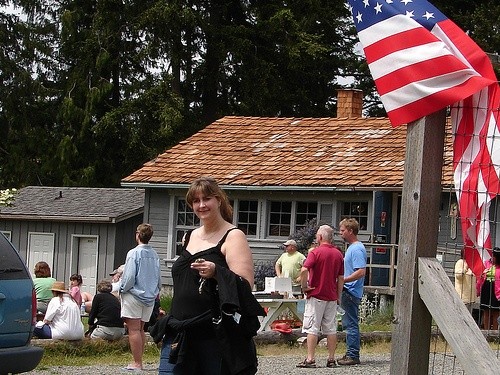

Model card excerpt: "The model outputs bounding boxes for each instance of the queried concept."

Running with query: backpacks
[272,322,292,334]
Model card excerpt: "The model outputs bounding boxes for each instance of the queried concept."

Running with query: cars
[0,229,44,375]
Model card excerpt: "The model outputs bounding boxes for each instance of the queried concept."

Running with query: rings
[203,271,204,274]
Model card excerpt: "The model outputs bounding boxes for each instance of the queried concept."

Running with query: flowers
[0,188,18,207]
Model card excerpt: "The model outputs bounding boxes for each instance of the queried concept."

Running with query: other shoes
[337,355,360,365]
[326,360,337,367]
[296,359,316,368]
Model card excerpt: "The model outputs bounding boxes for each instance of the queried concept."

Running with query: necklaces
[200,220,224,240]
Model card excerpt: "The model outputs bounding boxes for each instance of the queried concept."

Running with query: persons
[476,247,500,329]
[119,223,166,369]
[337,218,367,366]
[85,278,124,341]
[33,261,57,322]
[275,240,309,292]
[160,177,254,375]
[82,269,123,313]
[296,225,344,366]
[33,281,84,340]
[70,274,82,309]
[454,248,476,315]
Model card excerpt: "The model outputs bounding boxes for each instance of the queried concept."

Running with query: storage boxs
[265,277,292,299]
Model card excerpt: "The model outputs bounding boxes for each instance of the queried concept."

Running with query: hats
[283,240,298,247]
[46,281,69,292]
[109,269,118,276]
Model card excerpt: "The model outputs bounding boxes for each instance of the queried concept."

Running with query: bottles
[337,314,343,331]
[81,302,85,314]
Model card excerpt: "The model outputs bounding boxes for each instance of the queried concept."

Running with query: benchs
[35,312,89,322]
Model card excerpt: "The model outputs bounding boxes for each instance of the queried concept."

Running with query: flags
[350,0,500,294]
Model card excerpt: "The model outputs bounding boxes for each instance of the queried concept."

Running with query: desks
[257,298,306,330]
